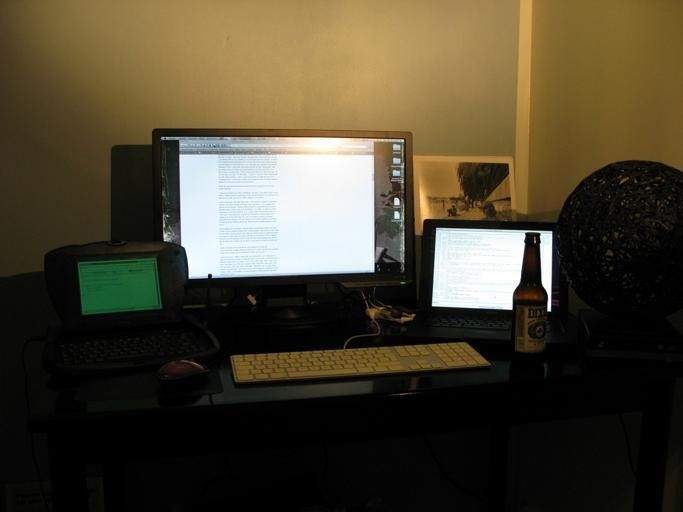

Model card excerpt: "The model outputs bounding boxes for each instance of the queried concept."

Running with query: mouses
[155,358,210,390]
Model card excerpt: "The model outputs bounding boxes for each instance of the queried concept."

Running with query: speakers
[111,145,154,241]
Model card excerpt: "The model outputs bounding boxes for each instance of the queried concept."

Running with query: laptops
[406,219,568,346]
[42,241,221,373]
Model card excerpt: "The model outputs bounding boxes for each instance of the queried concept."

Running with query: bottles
[512,231,549,364]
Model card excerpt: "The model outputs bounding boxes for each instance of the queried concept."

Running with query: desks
[38,266,683,512]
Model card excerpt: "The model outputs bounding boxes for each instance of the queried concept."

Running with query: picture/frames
[412,153,516,238]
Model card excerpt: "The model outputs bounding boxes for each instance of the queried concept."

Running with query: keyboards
[229,341,491,385]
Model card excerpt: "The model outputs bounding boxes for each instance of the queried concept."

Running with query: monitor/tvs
[151,128,416,327]
